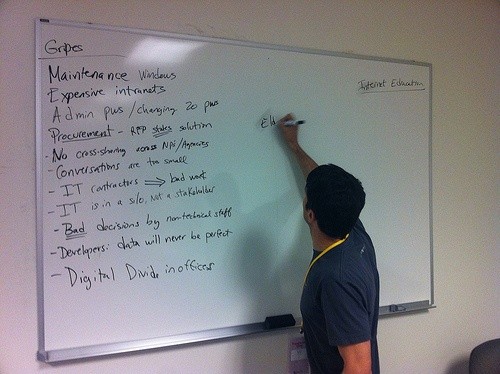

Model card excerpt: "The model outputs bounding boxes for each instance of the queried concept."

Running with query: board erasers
[263,314,296,330]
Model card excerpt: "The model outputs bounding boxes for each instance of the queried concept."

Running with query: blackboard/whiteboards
[34,16,437,364]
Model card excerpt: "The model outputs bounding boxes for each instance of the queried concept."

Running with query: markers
[277,118,305,128]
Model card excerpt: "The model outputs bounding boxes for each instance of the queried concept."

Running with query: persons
[275,114,381,374]
